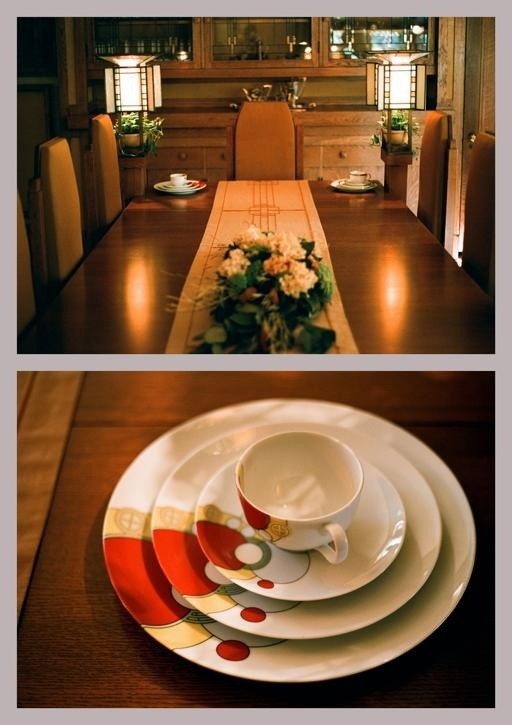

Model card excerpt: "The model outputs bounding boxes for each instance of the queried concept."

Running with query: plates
[149,419,444,643]
[329,178,377,192]
[100,394,479,685]
[153,180,208,195]
[193,450,408,605]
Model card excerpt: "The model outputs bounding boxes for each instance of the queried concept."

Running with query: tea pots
[241,84,273,101]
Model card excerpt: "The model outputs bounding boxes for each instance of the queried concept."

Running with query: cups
[170,173,187,186]
[349,171,371,183]
[233,430,366,567]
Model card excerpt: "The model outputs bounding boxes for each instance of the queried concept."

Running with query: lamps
[94,54,164,113]
[365,49,430,113]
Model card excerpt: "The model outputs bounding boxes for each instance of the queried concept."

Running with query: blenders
[280,76,307,108]
